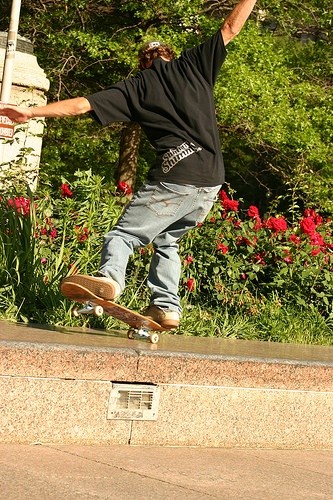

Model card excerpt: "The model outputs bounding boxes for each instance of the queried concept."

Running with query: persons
[0,0,258,327]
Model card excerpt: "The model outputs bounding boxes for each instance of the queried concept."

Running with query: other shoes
[142,304,182,328]
[60,275,122,301]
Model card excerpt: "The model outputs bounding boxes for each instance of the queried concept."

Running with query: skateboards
[61,282,172,344]
